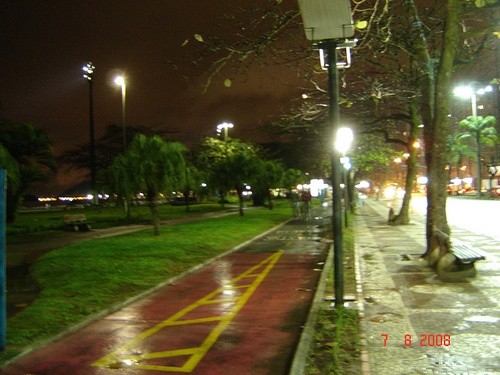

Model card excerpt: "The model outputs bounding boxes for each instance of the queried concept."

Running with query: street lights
[114,76,127,212]
[453,85,494,197]
[336,126,353,227]
[216,122,234,141]
[82,61,98,205]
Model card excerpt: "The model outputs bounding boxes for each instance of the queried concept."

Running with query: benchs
[433,225,486,283]
[62,213,97,232]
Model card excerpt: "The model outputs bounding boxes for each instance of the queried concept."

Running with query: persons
[290,189,312,221]
